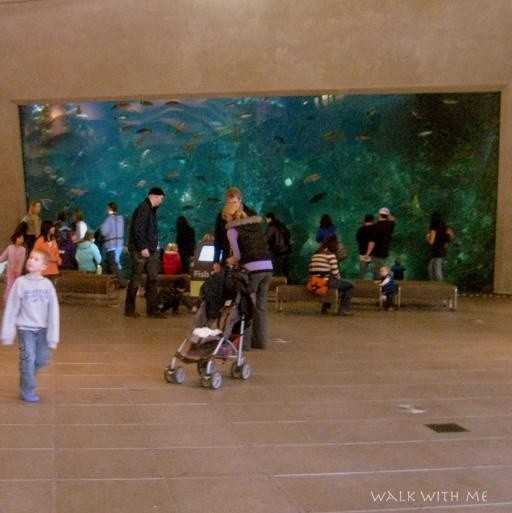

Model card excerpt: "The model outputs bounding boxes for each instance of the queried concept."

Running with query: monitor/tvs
[196,242,223,266]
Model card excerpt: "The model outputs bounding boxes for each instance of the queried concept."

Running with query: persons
[176,215,196,275]
[221,202,273,349]
[71,209,88,243]
[426,211,454,281]
[191,233,216,276]
[0,231,26,307]
[99,203,123,272]
[74,234,102,273]
[263,212,291,278]
[308,239,355,316]
[15,200,43,253]
[211,262,221,275]
[363,207,397,280]
[315,214,338,256]
[32,220,63,288]
[124,187,168,319]
[0,247,61,402]
[374,266,397,311]
[58,231,77,270]
[212,188,257,341]
[356,213,377,279]
[163,242,182,275]
[390,257,407,281]
[157,277,198,316]
[54,213,71,244]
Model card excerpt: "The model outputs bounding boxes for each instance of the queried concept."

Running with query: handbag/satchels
[336,240,348,259]
[305,276,328,296]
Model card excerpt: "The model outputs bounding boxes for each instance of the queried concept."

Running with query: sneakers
[147,311,167,319]
[337,310,353,316]
[320,309,328,314]
[19,392,39,402]
[124,311,140,318]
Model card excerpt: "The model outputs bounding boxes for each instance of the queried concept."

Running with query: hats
[378,207,390,215]
[148,187,165,196]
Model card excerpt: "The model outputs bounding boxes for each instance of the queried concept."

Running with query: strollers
[164,265,252,390]
